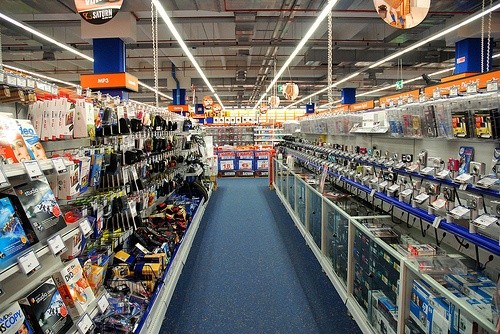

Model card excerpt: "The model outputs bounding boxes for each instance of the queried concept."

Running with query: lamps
[368,0,500,70]
[0,10,96,63]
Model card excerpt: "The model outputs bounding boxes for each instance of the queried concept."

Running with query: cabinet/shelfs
[270,90,500,334]
[1,64,216,334]
[199,123,297,178]
